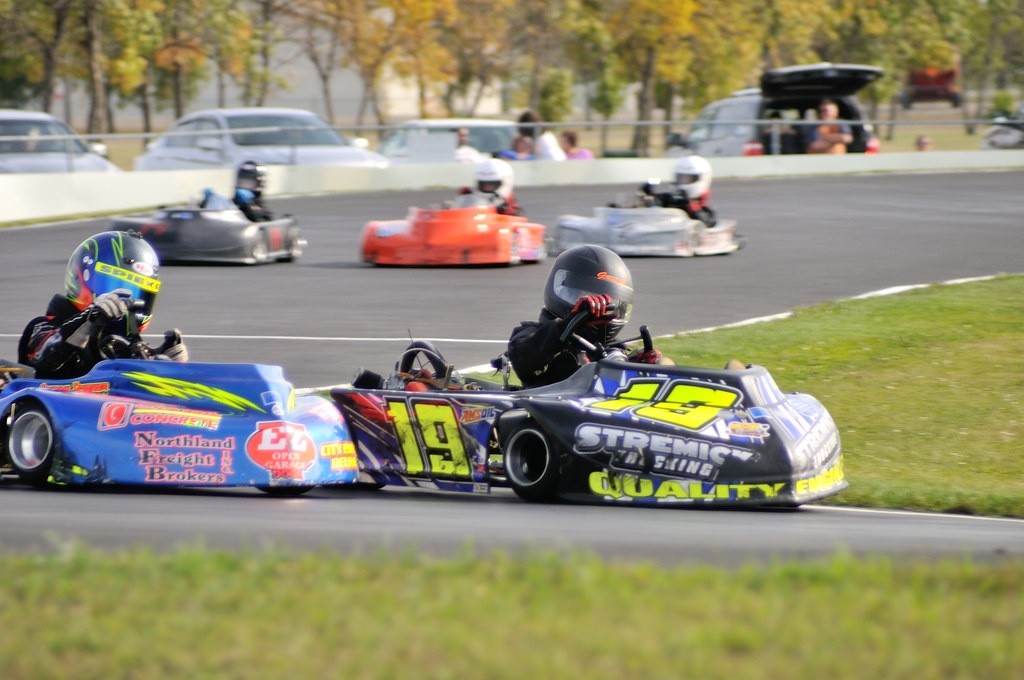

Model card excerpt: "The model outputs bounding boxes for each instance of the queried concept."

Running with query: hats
[662,132,687,147]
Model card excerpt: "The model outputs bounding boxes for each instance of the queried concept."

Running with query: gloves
[83,288,132,322]
[562,294,619,329]
[635,346,662,365]
[153,329,189,362]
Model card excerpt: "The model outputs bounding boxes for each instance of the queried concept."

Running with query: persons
[917,135,931,150]
[641,155,717,228]
[508,244,662,388]
[807,101,853,154]
[453,111,593,159]
[18,229,188,379]
[201,160,271,222]
[460,158,518,215]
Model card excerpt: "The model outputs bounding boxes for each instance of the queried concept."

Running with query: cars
[1,108,123,174]
[129,105,389,171]
[379,119,548,169]
[661,60,890,162]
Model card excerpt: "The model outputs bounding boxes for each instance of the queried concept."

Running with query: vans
[900,36,965,110]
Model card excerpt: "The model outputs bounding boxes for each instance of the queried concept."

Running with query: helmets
[63,227,163,332]
[234,159,267,204]
[472,157,517,206]
[542,243,634,343]
[671,156,713,199]
